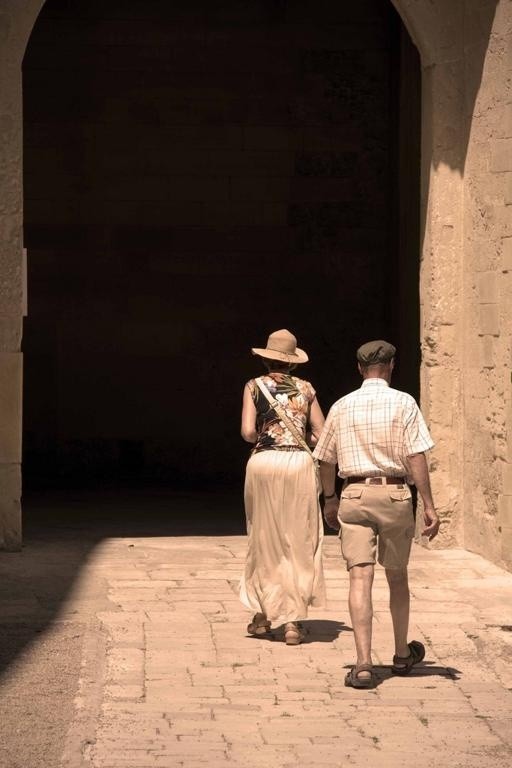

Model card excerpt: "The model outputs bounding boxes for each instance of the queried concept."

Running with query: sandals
[392,640,425,675]
[285,623,309,645]
[344,664,378,688]
[247,613,270,637]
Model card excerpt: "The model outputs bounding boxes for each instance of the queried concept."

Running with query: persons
[312,341,440,689]
[240,330,326,645]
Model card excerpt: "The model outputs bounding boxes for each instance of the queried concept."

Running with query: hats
[250,329,310,364]
[357,340,397,361]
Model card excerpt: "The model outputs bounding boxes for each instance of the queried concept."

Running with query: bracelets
[323,492,336,501]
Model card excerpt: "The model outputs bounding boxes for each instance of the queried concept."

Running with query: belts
[344,476,406,485]
